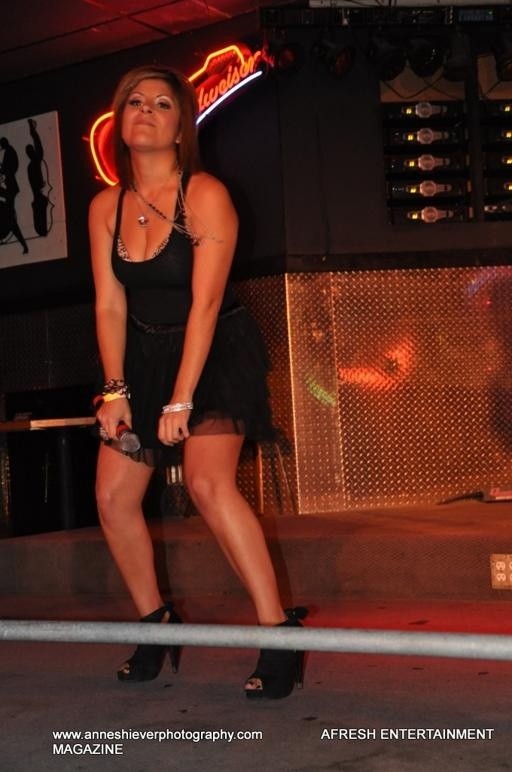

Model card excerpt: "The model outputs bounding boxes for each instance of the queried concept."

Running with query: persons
[86,67,309,698]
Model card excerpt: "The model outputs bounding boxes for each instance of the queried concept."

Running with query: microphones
[92,396,141,454]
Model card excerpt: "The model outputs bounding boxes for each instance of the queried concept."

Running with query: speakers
[5,385,162,536]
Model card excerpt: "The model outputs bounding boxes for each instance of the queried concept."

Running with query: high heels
[244,606,309,700]
[117,601,183,683]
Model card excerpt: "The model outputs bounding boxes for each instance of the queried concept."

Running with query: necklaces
[129,164,224,247]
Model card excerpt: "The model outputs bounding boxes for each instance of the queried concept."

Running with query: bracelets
[102,391,127,401]
[160,401,193,414]
[102,378,128,396]
